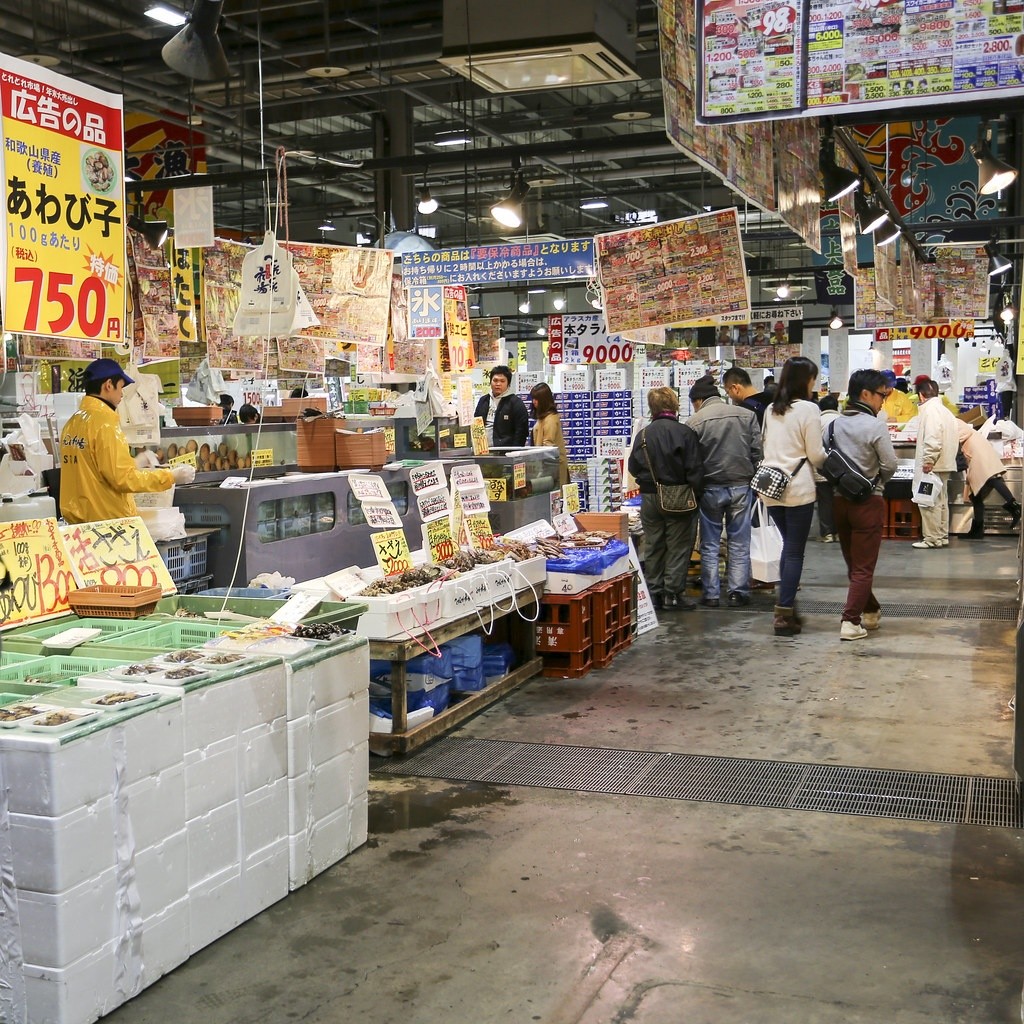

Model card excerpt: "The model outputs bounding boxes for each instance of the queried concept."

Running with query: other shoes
[697,598,719,607]
[833,533,840,542]
[650,595,663,608]
[816,534,833,543]
[662,592,697,609]
[727,592,749,606]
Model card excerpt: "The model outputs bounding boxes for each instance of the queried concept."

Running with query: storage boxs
[453,360,732,514]
[816,478,921,540]
[341,400,380,414]
[0,512,639,1024]
[137,414,560,588]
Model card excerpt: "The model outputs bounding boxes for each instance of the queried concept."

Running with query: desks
[370,580,543,755]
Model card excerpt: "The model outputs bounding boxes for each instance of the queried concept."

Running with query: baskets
[0,585,244,707]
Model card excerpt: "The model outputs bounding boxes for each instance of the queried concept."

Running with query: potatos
[156,440,251,470]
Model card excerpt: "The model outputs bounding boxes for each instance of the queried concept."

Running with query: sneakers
[860,609,881,630]
[840,621,868,641]
[942,539,949,545]
[912,540,942,548]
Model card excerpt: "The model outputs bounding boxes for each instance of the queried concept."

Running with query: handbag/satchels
[656,484,698,513]
[750,498,784,583]
[749,465,789,500]
[911,467,943,507]
[822,447,872,504]
[955,452,968,472]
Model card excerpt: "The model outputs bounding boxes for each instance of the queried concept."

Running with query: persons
[716,325,734,346]
[291,388,308,398]
[685,374,762,608]
[912,380,960,549]
[474,366,529,447]
[628,386,703,610]
[240,405,263,424]
[759,356,829,634]
[735,325,751,345]
[530,383,569,486]
[823,369,898,641]
[813,396,841,542]
[881,370,958,423]
[665,328,699,348]
[220,394,238,424]
[724,367,774,423]
[751,321,789,346]
[60,359,194,522]
[956,418,1022,539]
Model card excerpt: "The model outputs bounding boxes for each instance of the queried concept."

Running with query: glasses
[873,391,886,399]
[725,383,738,398]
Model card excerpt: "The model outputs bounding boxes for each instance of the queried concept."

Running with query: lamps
[417,174,438,214]
[983,235,1013,276]
[468,298,568,314]
[491,172,532,228]
[126,191,167,250]
[830,311,843,330]
[161,0,229,80]
[317,218,336,232]
[969,115,1018,195]
[819,127,902,246]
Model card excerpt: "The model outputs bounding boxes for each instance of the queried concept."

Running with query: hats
[82,358,135,388]
[688,375,722,398]
[913,375,930,385]
[881,369,897,388]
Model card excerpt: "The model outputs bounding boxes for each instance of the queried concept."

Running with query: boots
[958,519,984,538]
[1002,498,1022,529]
[773,605,801,636]
